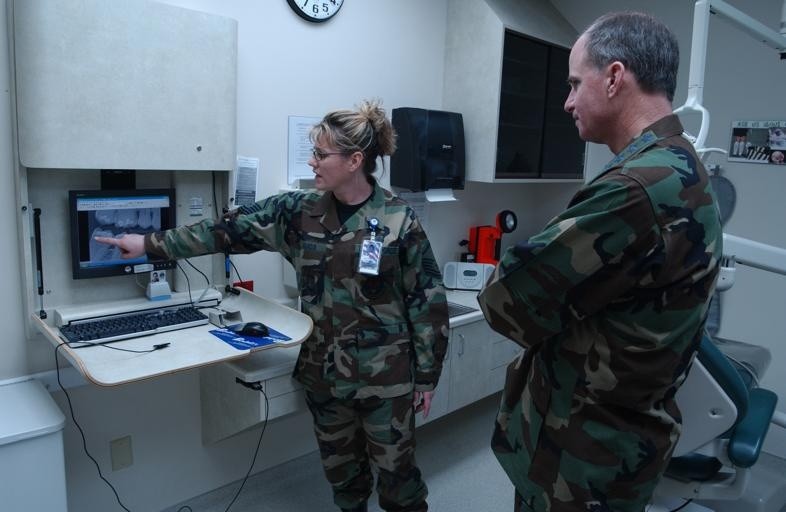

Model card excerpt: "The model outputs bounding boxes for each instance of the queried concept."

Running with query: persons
[94,98,450,511]
[732,127,786,164]
[476,11,723,511]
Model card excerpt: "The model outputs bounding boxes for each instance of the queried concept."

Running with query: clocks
[287,0,344,23]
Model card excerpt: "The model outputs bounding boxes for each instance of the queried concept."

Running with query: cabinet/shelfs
[491,27,587,184]
[414,320,519,430]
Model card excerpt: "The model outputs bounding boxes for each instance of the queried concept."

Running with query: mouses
[234,321,268,338]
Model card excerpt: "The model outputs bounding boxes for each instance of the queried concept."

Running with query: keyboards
[57,305,209,348]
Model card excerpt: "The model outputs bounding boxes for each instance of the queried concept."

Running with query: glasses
[311,149,353,161]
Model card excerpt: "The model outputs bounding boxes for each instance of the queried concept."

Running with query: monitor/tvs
[67,187,177,279]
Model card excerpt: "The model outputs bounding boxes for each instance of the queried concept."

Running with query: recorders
[443,262,496,291]
